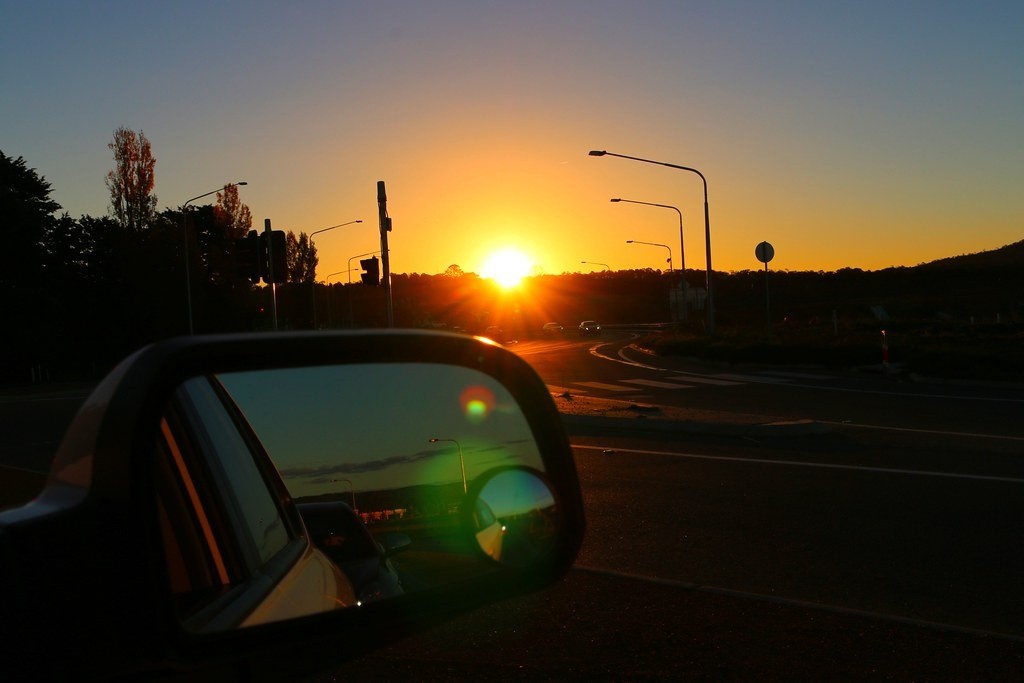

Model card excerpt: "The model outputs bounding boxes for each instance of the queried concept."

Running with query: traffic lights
[237,236,265,279]
[360,256,380,287]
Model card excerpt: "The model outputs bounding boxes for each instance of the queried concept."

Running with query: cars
[543,323,566,337]
[299,502,411,605]
[579,321,602,338]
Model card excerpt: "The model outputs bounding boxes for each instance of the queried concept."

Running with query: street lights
[310,220,363,330]
[185,181,249,335]
[429,438,467,496]
[330,479,356,511]
[626,240,673,323]
[609,198,687,322]
[588,150,725,339]
